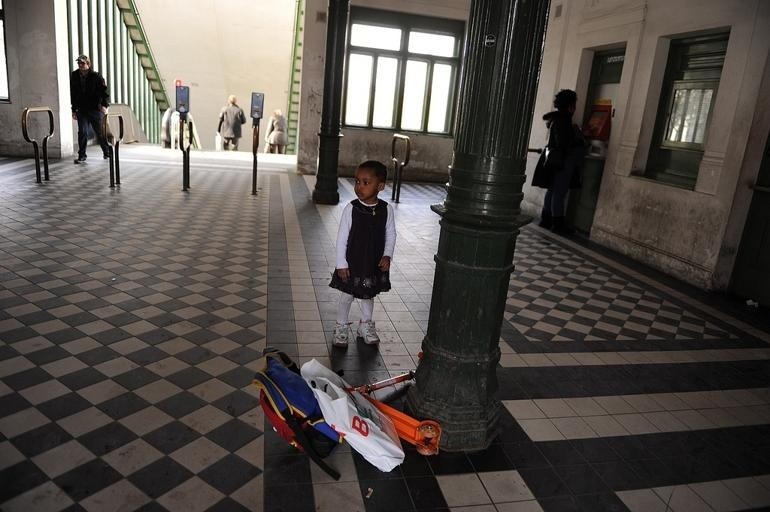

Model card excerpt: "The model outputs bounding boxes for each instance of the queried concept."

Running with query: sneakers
[357,319,380,345]
[333,321,350,348]
[103,150,110,159]
[74,158,87,164]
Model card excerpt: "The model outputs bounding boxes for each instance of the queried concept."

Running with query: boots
[540,209,573,236]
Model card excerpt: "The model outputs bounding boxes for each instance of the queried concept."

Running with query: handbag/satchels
[215,132,222,151]
[542,144,573,173]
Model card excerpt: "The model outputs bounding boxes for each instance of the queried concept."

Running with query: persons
[217,94,246,150]
[264,109,290,153]
[70,54,113,164]
[537,87,597,238]
[326,160,398,349]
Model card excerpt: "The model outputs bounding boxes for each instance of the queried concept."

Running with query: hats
[76,55,89,64]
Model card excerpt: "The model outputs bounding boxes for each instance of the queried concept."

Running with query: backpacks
[254,346,344,458]
